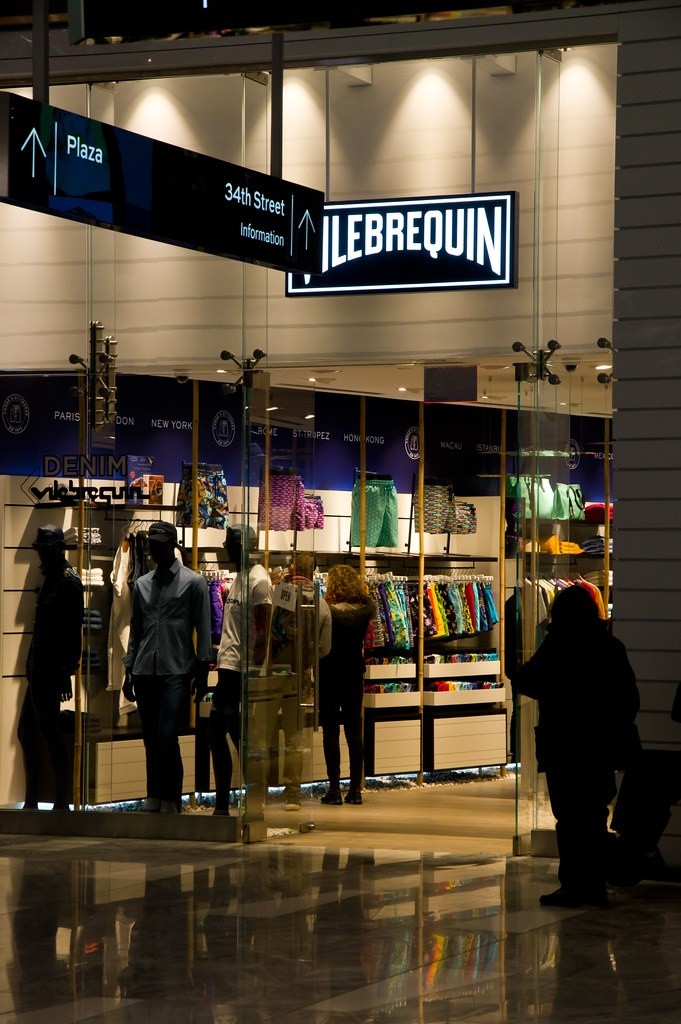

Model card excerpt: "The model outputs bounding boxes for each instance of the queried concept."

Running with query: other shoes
[344,789,364,804]
[284,793,301,810]
[321,789,343,805]
[540,888,581,908]
[580,890,610,906]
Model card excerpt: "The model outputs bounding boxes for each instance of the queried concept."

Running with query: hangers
[525,562,584,581]
[189,560,495,583]
[119,516,158,535]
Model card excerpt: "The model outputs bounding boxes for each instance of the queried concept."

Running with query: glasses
[32,539,62,551]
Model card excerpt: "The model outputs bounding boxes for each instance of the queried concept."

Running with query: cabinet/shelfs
[30,501,186,737]
[201,657,506,707]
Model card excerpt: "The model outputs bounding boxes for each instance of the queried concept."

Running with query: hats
[146,522,177,544]
[35,525,64,550]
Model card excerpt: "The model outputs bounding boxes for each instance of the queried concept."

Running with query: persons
[16,521,274,814]
[510,587,640,906]
[258,553,376,814]
